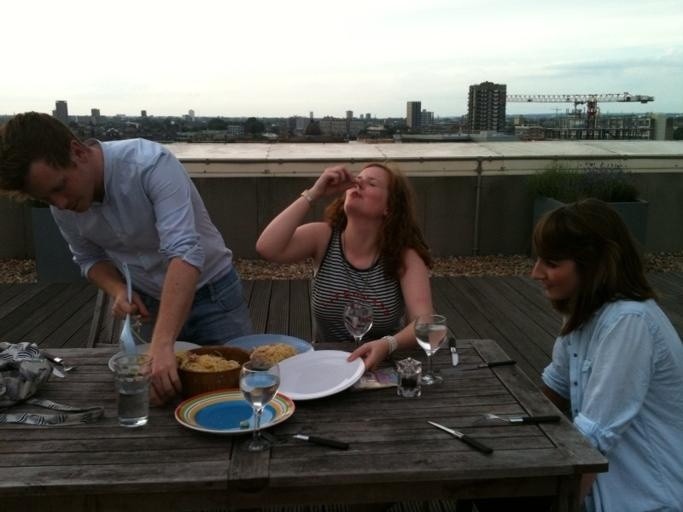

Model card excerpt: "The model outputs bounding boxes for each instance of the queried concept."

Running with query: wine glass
[415,312,450,385]
[239,361,280,453]
[344,303,373,350]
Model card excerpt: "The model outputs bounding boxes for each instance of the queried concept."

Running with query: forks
[473,413,561,429]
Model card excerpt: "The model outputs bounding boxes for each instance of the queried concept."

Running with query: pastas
[250,344,297,364]
[174,351,241,372]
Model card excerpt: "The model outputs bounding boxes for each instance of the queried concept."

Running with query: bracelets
[301,189,315,208]
[381,335,397,357]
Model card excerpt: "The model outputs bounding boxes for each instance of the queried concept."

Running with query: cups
[114,353,153,426]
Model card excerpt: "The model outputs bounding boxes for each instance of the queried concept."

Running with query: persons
[530,198,683,511]
[0,112,253,407]
[253,164,434,373]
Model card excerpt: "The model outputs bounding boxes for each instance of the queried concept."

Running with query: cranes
[506,92,655,115]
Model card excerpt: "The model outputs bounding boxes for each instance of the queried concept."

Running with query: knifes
[449,337,459,366]
[425,420,492,452]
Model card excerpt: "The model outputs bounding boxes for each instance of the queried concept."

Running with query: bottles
[397,359,422,400]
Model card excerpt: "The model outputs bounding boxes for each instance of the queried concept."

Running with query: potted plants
[530,152,652,263]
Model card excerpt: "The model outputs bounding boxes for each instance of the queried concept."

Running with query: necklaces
[343,229,380,296]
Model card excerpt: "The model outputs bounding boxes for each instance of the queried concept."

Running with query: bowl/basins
[176,346,249,396]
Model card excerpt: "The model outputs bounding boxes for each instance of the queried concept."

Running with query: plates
[222,332,312,371]
[268,347,366,399]
[107,339,203,374]
[175,390,295,436]
